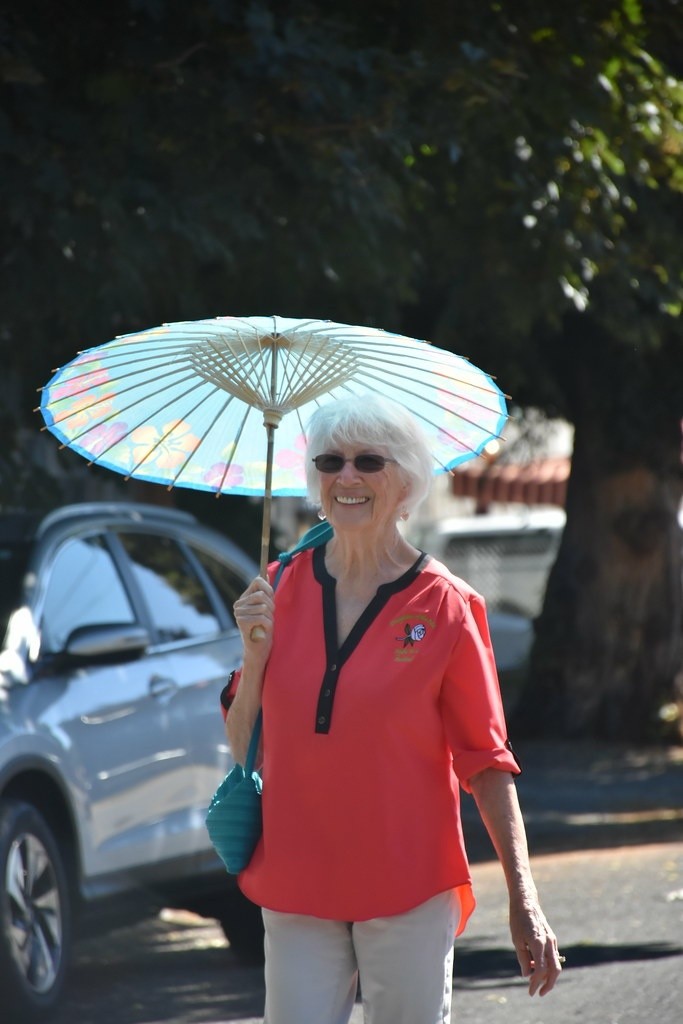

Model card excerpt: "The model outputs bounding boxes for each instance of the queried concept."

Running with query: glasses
[312,453,398,473]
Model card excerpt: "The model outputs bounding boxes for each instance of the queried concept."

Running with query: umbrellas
[32,315,516,643]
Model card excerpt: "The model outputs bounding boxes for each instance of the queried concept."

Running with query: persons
[219,393,566,1024]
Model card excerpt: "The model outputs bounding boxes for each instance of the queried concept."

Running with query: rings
[558,956,565,963]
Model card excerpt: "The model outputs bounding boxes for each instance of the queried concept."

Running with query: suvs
[1,499,268,1024]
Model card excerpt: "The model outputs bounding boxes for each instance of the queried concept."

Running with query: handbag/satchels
[205,762,263,876]
